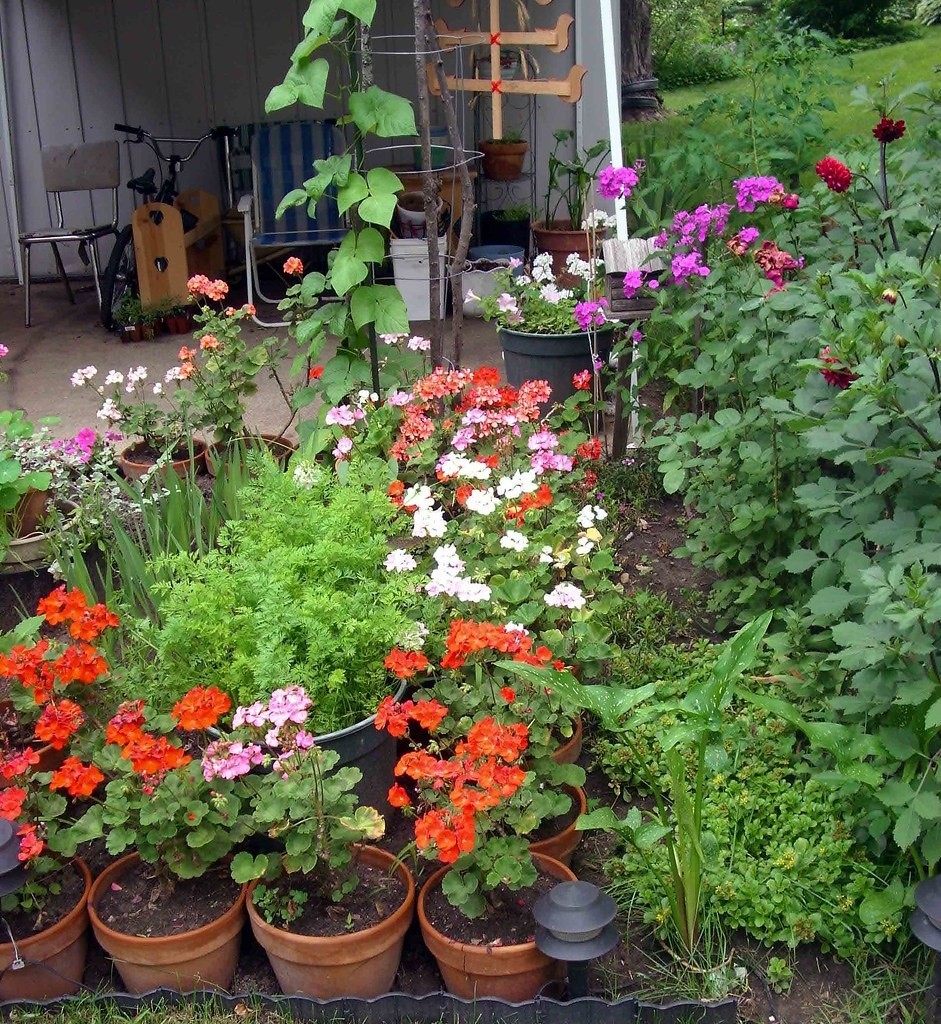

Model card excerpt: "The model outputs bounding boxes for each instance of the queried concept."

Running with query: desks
[386,163,479,254]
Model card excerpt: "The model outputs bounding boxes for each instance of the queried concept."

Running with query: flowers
[0,205,631,923]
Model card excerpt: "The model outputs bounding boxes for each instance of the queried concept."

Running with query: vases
[0,845,93,1000]
[204,434,293,481]
[469,244,526,296]
[442,712,585,765]
[494,322,614,434]
[245,842,417,999]
[417,852,578,1002]
[0,698,68,793]
[120,436,209,484]
[88,849,249,993]
[7,487,56,539]
[0,503,82,696]
[496,783,589,867]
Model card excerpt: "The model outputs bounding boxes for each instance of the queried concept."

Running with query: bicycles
[99,123,244,330]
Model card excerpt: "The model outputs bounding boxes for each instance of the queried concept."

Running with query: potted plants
[159,291,180,334]
[530,128,611,287]
[173,304,190,334]
[443,257,511,320]
[140,301,160,341]
[149,447,421,833]
[186,307,195,330]
[154,313,162,336]
[112,288,133,344]
[393,190,444,238]
[125,299,143,342]
[481,199,544,260]
[478,124,528,182]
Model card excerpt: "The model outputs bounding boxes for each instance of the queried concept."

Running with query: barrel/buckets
[470,245,525,287]
[390,233,448,321]
[412,126,449,172]
[397,190,443,239]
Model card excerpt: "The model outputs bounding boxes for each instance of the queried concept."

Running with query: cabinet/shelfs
[132,187,225,309]
[468,48,541,248]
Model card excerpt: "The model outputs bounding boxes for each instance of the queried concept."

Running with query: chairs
[17,140,126,329]
[237,122,355,329]
